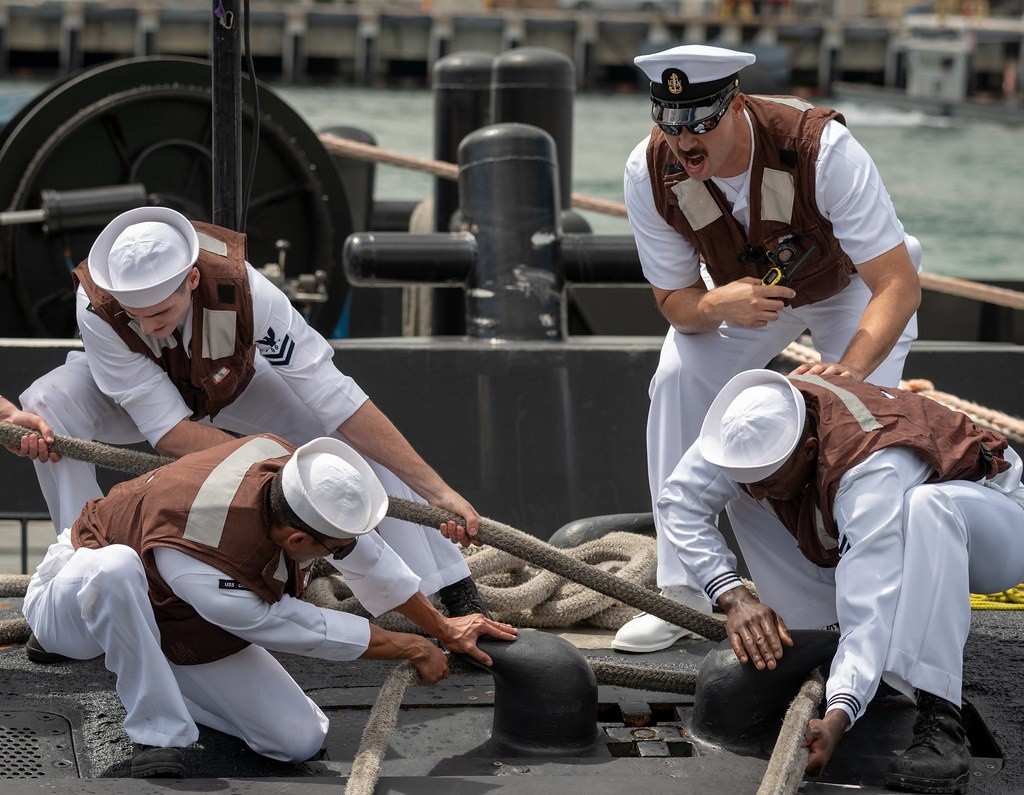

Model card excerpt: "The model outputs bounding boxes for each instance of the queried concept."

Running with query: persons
[18,207,494,659]
[24,433,518,778]
[0,394,62,463]
[655,368,1024,795]
[611,45,922,652]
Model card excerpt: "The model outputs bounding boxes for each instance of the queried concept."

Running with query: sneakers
[195,721,248,753]
[131,740,187,779]
[439,576,492,621]
[26,633,61,663]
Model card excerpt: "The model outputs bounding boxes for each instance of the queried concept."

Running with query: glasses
[657,85,740,135]
[289,522,360,560]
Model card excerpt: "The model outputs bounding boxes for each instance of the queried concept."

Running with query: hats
[701,368,807,484]
[633,44,756,126]
[88,207,200,309]
[281,437,389,539]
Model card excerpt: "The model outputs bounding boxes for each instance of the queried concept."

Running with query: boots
[885,686,971,794]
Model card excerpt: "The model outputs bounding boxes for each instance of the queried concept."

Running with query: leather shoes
[610,586,714,650]
